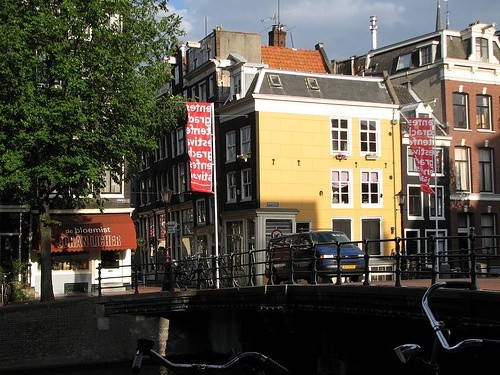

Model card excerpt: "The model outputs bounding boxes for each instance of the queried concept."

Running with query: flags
[182,101,213,194]
[408,116,436,195]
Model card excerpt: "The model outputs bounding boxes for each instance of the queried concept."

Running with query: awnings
[32,213,138,255]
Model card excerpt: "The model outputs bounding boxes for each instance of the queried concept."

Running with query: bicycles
[206,251,248,289]
[385,278,500,375]
[130,275,301,375]
[1,272,19,306]
[175,251,213,291]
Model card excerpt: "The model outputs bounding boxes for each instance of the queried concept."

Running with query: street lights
[159,188,181,295]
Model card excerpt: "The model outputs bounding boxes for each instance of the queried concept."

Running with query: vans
[266,229,366,286]
